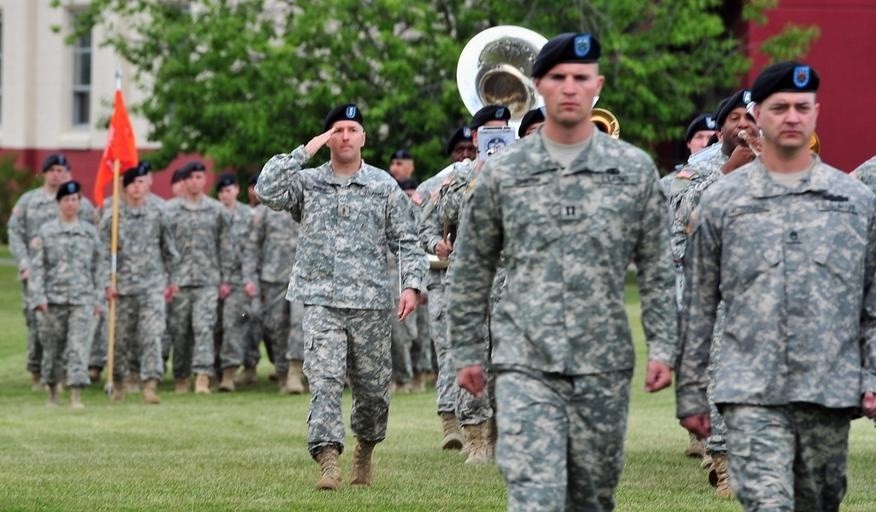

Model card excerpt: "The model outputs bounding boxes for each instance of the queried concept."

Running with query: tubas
[425,25,550,268]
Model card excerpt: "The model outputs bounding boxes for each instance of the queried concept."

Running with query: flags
[92,91,140,211]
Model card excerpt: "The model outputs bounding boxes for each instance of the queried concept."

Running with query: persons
[847,152,876,196]
[159,162,309,395]
[97,167,181,405]
[661,89,761,494]
[27,180,106,411]
[386,178,449,394]
[443,33,682,511]
[678,58,876,511]
[89,160,166,395]
[389,148,413,179]
[408,106,546,467]
[255,102,430,491]
[7,153,95,392]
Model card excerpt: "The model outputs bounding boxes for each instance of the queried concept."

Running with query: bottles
[534,33,599,77]
[396,180,418,189]
[391,149,410,158]
[57,181,82,201]
[322,103,363,129]
[445,103,546,159]
[43,155,69,172]
[685,62,820,149]
[123,160,263,188]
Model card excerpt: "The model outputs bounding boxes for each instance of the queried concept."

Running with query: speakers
[685,432,705,458]
[440,411,463,452]
[314,447,342,490]
[269,362,438,395]
[350,442,375,488]
[464,421,489,465]
[711,452,736,497]
[32,368,255,408]
